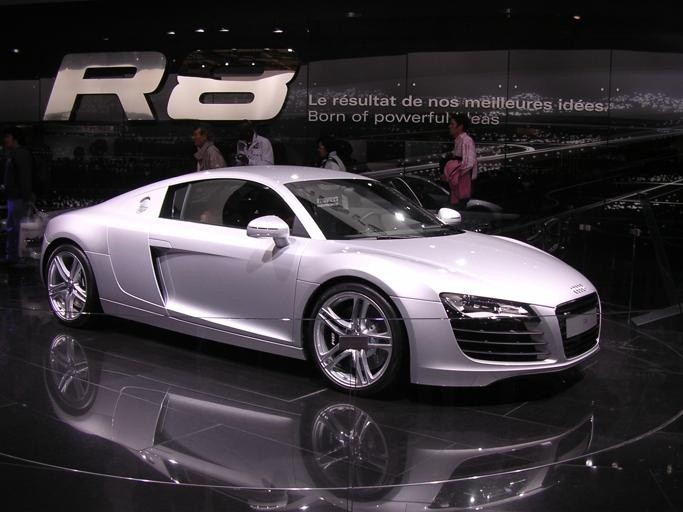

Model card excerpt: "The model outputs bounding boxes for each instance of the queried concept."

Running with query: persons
[1,127,34,265]
[234,120,275,166]
[438,113,478,210]
[190,127,227,172]
[316,136,346,173]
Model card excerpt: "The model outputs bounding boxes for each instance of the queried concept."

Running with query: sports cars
[44,323,595,512]
[37,164,600,397]
[393,174,504,219]
